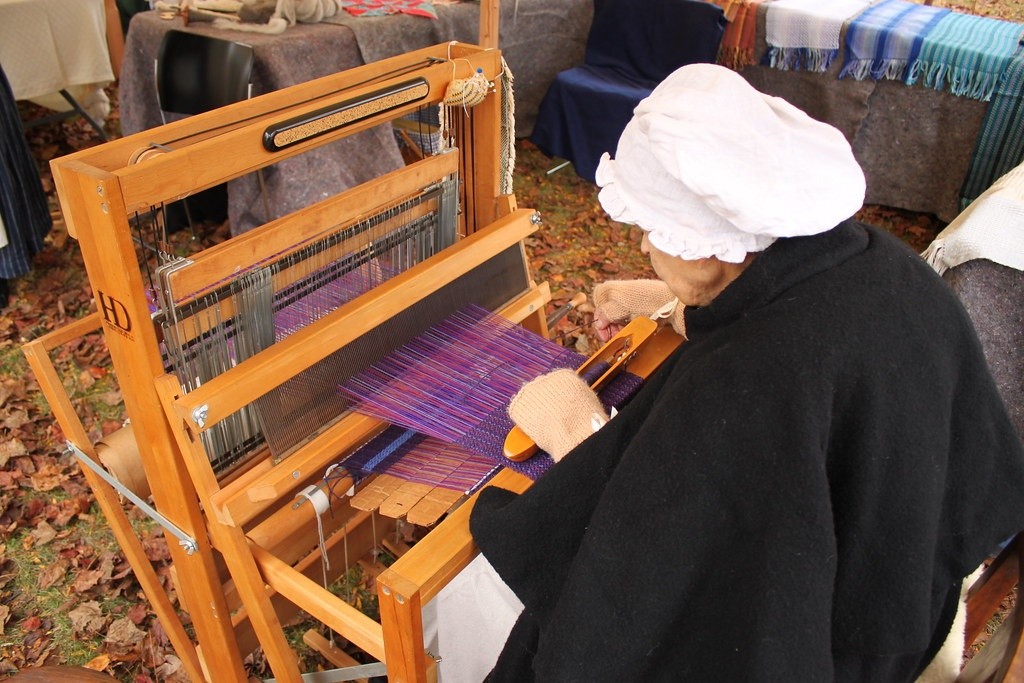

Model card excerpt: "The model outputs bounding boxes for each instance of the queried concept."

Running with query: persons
[435,61,1024,683]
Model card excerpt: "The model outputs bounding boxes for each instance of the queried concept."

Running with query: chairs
[118,27,276,266]
[956,526,1024,683]
[531,0,731,189]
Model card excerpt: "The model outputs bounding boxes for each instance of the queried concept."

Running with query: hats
[595,61,868,263]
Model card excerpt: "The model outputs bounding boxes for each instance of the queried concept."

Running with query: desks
[909,161,1024,444]
[126,0,482,230]
[703,0,1023,221]
